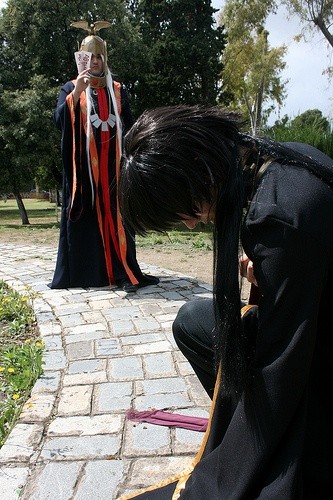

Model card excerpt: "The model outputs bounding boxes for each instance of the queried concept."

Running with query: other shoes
[120,280,141,292]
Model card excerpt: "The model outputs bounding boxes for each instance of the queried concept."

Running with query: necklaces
[88,87,116,132]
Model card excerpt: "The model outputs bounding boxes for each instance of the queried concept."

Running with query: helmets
[67,20,111,89]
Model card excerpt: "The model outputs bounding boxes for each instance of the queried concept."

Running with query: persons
[114,103,333,500]
[46,18,160,291]
